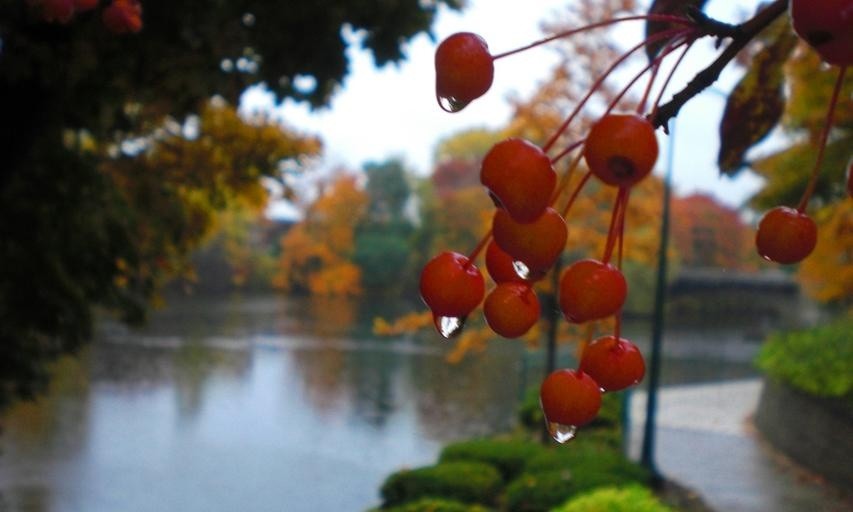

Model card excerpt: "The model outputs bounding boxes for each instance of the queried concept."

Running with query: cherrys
[436,31,494,105]
[754,204,814,265]
[417,116,659,428]
